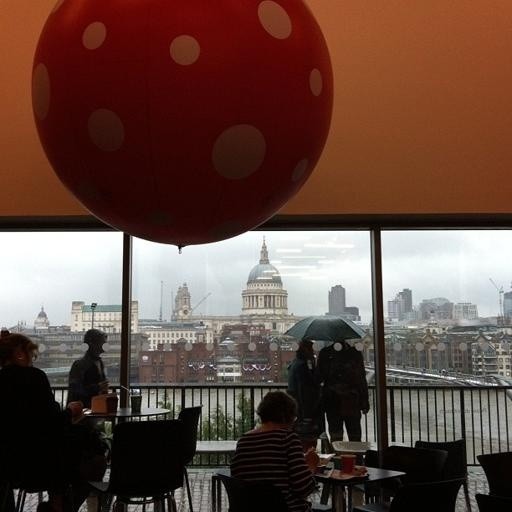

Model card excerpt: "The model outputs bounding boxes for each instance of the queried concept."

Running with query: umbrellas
[284,312,368,348]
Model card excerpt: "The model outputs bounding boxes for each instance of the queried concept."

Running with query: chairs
[1,408,205,512]
[207,433,511,512]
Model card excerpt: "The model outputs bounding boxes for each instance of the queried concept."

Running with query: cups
[131,395,142,414]
[340,454,356,474]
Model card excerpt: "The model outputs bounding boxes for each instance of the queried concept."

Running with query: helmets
[84,330,107,342]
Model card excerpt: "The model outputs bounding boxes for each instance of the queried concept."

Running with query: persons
[65,328,110,497]
[287,340,326,455]
[230,391,331,512]
[315,337,370,456]
[0,328,107,512]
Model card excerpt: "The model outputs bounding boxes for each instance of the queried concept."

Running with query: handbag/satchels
[293,415,323,433]
